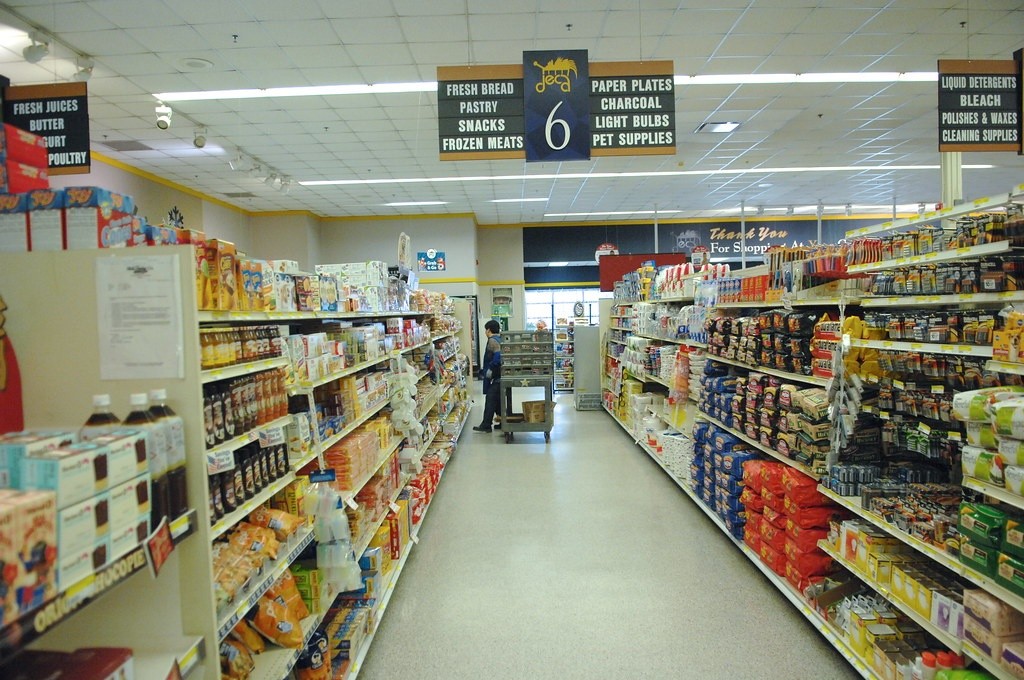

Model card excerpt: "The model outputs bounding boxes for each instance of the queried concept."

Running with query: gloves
[485,368,493,379]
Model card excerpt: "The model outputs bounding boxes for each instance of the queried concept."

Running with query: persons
[535,320,548,333]
[472,320,504,433]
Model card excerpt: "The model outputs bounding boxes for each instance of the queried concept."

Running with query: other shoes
[473,425,493,433]
[494,422,501,429]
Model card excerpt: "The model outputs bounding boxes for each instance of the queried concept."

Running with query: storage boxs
[521,400,556,423]
[0,123,418,680]
[835,518,1024,680]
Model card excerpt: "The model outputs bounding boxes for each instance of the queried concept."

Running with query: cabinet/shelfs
[601,238,1024,680]
[500,331,554,431]
[0,245,474,680]
[556,324,600,411]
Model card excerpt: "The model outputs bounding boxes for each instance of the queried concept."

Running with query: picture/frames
[491,287,514,317]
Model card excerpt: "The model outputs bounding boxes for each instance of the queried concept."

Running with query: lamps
[230,146,245,169]
[264,167,277,185]
[155,106,172,129]
[190,124,207,149]
[24,30,50,65]
[279,175,292,192]
[248,160,263,177]
[68,55,95,83]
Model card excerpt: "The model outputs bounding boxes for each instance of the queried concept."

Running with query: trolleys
[499,330,556,444]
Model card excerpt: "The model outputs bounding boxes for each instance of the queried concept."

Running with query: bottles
[148,387,189,521]
[200,324,283,370]
[78,394,122,442]
[204,367,289,449]
[689,314,707,343]
[694,276,715,306]
[118,393,172,534]
